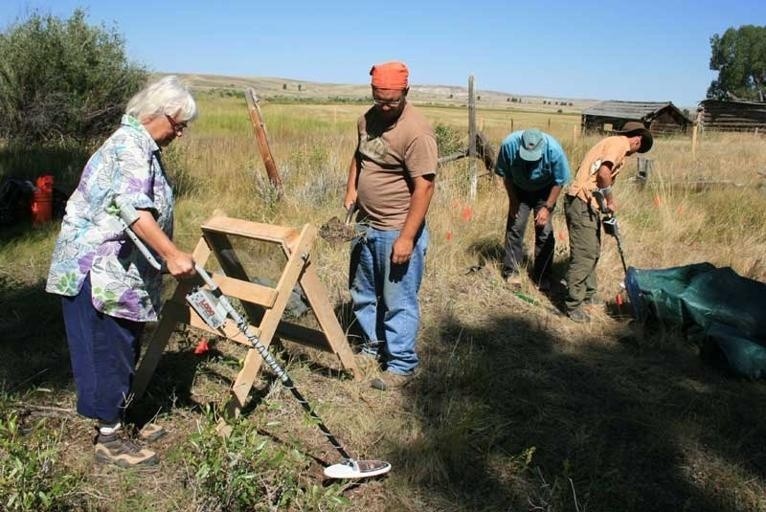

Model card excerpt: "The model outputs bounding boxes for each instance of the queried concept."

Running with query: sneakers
[585,297,606,304]
[370,369,418,389]
[536,280,548,291]
[568,309,589,323]
[508,277,521,288]
[94,423,166,467]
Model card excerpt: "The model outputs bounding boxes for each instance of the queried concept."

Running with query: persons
[559,121,652,322]
[493,128,570,291]
[343,61,437,390]
[45,74,197,468]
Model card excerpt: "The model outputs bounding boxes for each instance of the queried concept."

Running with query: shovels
[319,188,361,245]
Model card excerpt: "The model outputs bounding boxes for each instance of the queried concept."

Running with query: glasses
[370,91,402,108]
[165,113,187,130]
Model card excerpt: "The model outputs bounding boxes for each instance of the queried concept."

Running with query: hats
[370,63,409,89]
[613,121,653,154]
[518,128,545,162]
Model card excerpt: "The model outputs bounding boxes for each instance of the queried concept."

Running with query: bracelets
[543,204,552,212]
[601,187,615,196]
